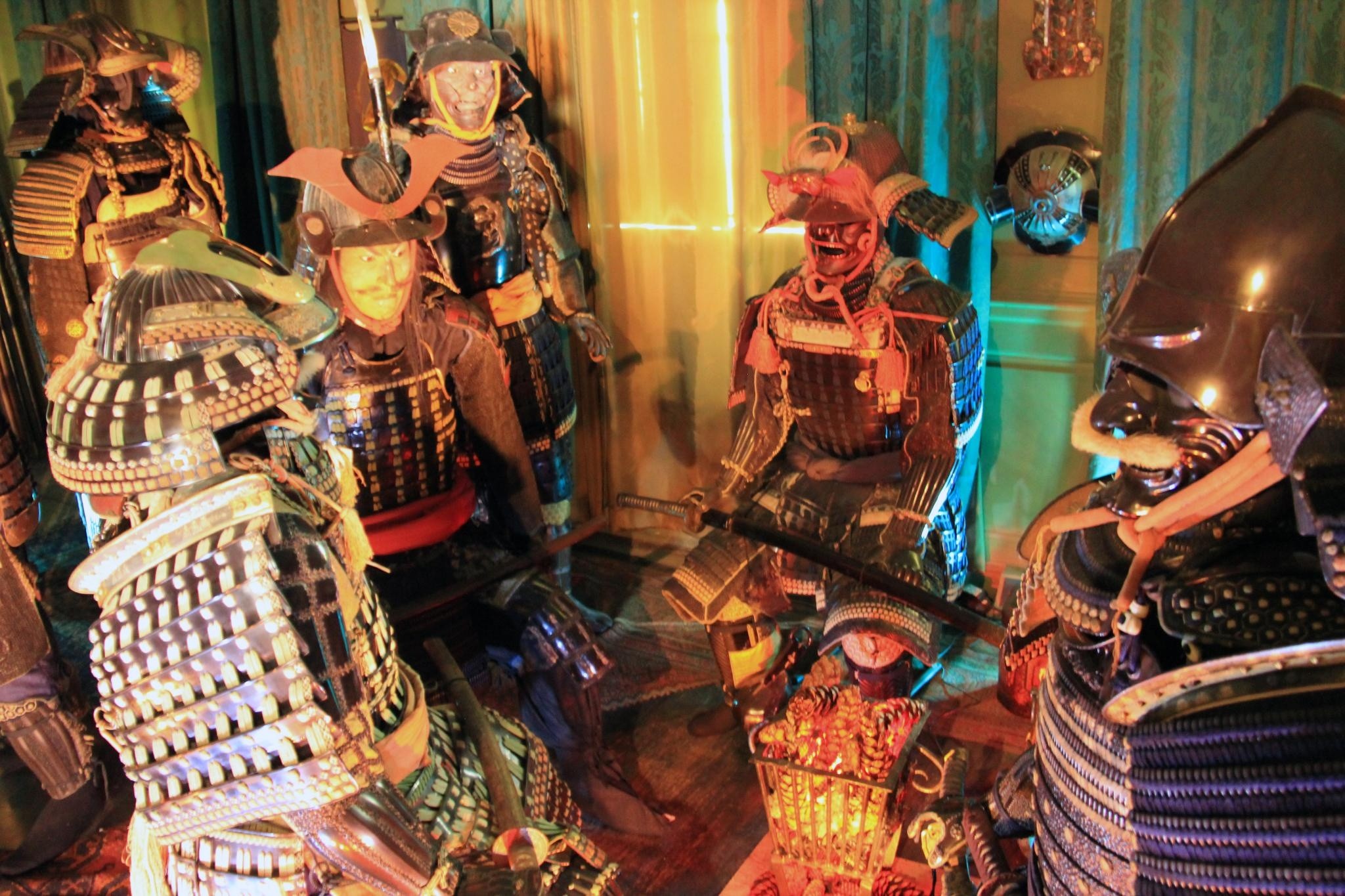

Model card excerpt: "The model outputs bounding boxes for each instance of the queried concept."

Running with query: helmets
[396,8,531,126]
[761,114,977,249]
[41,228,337,494]
[5,11,205,158]
[268,135,466,292]
[1106,86,1342,432]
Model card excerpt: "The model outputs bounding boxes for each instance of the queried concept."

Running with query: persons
[14,5,1345,896]
